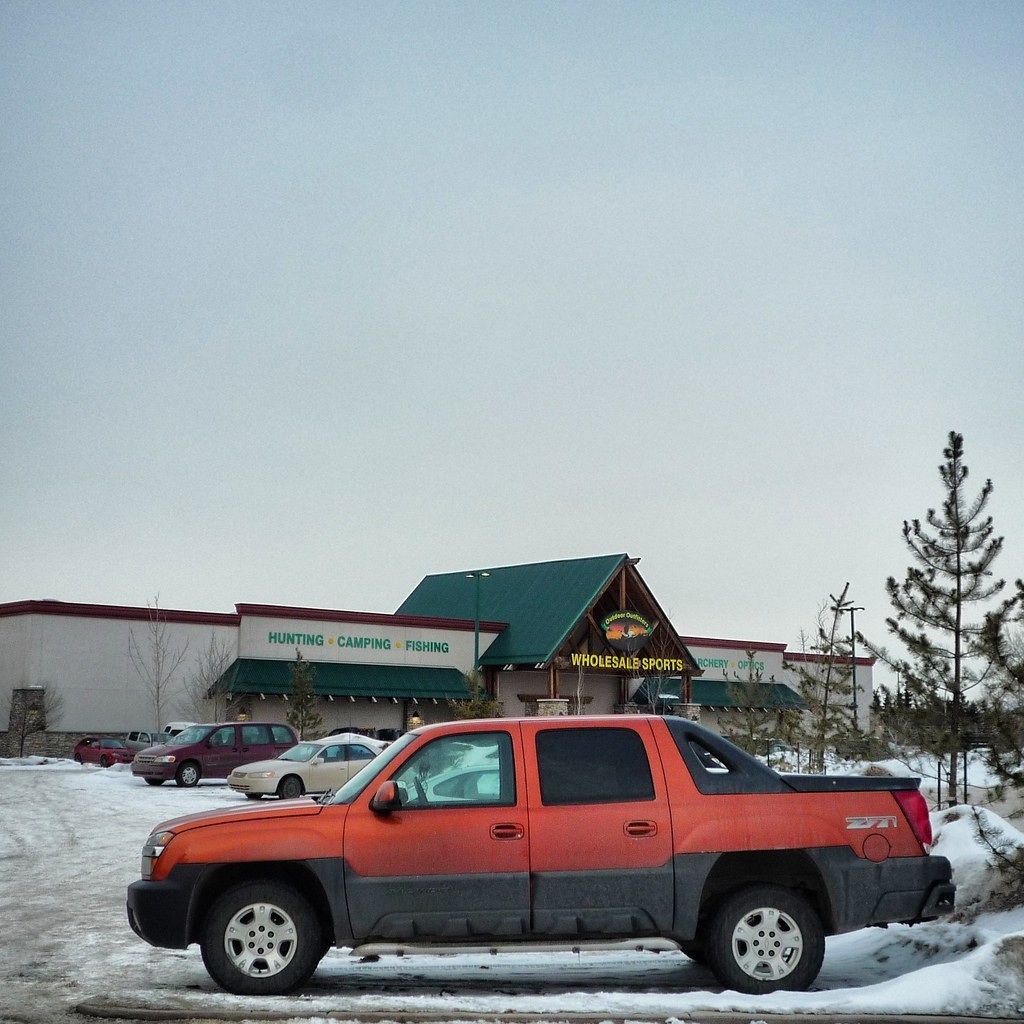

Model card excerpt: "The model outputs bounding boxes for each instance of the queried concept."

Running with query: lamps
[412,710,421,723]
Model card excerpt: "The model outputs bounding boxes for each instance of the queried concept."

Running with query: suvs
[130,721,301,788]
[125,729,177,753]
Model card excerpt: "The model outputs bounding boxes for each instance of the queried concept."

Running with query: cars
[74,734,135,768]
[226,738,501,808]
[125,713,957,1000]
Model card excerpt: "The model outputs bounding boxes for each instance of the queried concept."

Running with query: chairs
[245,737,251,743]
[214,732,222,745]
[277,736,286,743]
[227,733,236,745]
[255,734,266,744]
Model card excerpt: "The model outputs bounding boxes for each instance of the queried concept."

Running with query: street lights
[465,573,492,703]
[838,606,866,729]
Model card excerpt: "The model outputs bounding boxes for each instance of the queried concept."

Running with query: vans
[163,719,206,743]
